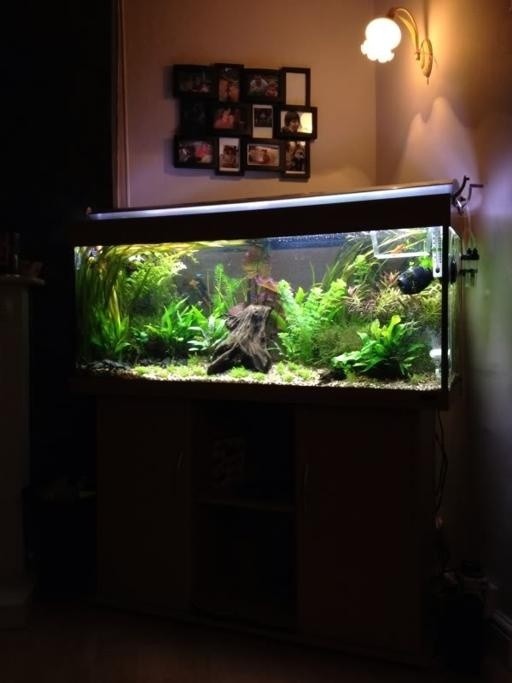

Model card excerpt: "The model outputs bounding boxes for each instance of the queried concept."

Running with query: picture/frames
[164,58,325,181]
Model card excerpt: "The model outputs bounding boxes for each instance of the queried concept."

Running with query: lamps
[359,4,434,84]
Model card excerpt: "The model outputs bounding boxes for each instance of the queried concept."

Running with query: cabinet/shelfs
[65,370,476,674]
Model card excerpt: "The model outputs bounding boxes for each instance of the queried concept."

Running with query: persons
[177,65,307,171]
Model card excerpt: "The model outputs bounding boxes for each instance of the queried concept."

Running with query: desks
[0,273,48,634]
